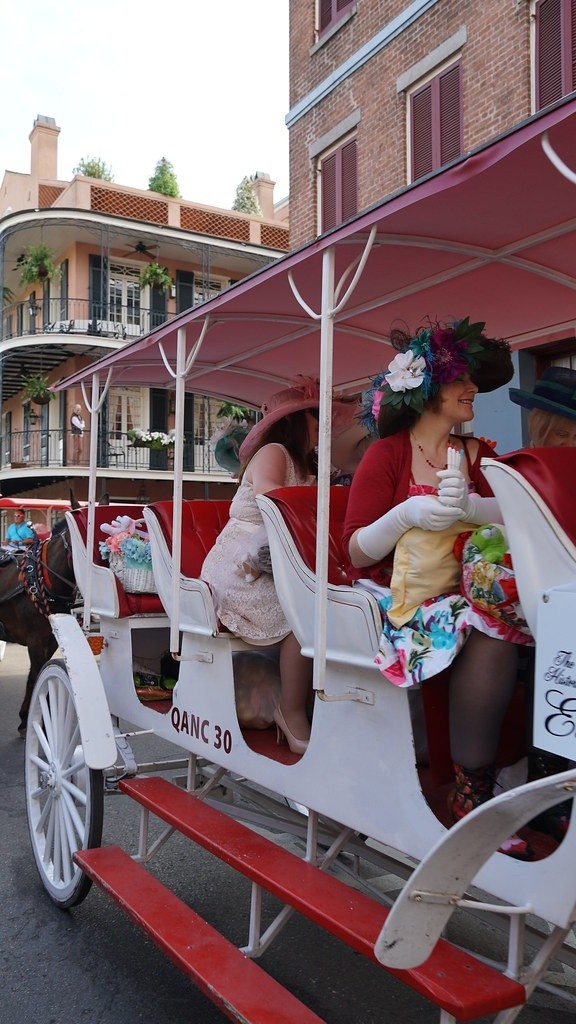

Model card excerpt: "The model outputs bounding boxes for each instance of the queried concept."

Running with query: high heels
[271,702,310,755]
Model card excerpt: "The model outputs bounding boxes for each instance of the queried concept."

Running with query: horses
[0,487,110,740]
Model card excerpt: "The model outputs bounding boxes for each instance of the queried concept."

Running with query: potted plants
[137,261,174,296]
[20,373,55,404]
[8,242,66,286]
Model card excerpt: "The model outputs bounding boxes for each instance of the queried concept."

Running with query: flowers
[355,316,512,432]
[127,428,174,450]
[99,531,152,566]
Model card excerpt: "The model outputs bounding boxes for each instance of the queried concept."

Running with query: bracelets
[18,541,22,545]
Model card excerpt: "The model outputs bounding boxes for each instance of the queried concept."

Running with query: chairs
[102,439,126,469]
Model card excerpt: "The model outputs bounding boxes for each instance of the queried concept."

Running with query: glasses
[304,408,319,421]
[13,514,25,519]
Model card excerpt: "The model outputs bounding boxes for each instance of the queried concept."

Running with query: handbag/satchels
[453,523,535,639]
[159,648,182,693]
[387,521,484,630]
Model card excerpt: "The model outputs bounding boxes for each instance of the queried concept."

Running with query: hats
[509,366,576,421]
[351,314,515,440]
[238,373,361,465]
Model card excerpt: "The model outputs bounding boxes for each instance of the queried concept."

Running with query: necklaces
[412,432,451,470]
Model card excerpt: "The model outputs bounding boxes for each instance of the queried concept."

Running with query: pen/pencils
[447,443,465,470]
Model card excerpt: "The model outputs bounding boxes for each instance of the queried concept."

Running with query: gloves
[435,469,506,526]
[256,546,273,576]
[356,495,467,560]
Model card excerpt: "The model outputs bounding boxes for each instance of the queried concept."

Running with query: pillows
[387,511,482,628]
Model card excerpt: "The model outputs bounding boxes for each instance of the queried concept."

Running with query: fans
[120,240,160,260]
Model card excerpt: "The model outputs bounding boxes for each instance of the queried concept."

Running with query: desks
[127,445,150,470]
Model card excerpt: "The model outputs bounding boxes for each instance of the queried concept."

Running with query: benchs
[479,445,576,761]
[65,503,168,621]
[143,497,239,643]
[254,483,419,674]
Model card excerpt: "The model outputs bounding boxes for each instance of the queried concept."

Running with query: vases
[149,446,169,470]
[134,438,152,448]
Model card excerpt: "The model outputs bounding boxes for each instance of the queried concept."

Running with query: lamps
[27,304,42,316]
[28,408,44,425]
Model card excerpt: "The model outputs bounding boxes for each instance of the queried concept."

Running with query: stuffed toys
[471,525,509,564]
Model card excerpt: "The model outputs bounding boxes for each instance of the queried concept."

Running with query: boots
[444,762,532,860]
[524,752,572,842]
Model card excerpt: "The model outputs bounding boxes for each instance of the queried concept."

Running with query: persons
[198,376,335,755]
[0,509,36,553]
[509,366,576,448]
[70,404,85,465]
[341,317,576,860]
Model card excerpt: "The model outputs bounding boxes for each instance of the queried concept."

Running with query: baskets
[99,515,160,594]
